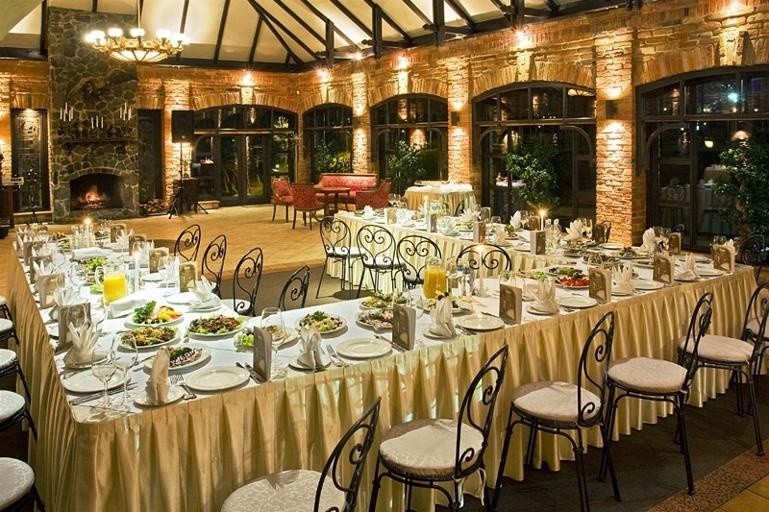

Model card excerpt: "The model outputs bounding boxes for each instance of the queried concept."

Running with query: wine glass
[110,333,139,408]
[43,238,106,336]
[576,218,593,236]
[261,307,288,370]
[92,347,117,411]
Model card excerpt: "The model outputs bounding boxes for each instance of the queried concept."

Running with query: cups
[499,271,516,286]
[652,226,671,238]
[712,235,726,246]
[423,257,447,298]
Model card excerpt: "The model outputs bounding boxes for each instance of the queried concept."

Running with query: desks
[0,185,15,229]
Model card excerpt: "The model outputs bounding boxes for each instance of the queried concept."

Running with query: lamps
[733,122,749,141]
[83,1,192,65]
[704,122,714,149]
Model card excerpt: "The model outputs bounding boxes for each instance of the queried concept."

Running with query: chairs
[0,288,45,512]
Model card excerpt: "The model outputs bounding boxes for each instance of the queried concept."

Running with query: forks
[456,325,476,336]
[244,361,265,382]
[170,376,190,400]
[327,344,349,368]
[175,372,196,399]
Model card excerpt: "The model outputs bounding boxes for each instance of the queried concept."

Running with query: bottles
[447,257,474,298]
[544,217,561,248]
[70,224,95,248]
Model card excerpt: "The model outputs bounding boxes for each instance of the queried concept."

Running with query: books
[14,230,91,351]
[649,253,673,285]
[110,224,199,293]
[587,268,613,305]
[711,242,731,274]
[251,324,274,382]
[381,200,608,256]
[498,284,524,328]
[663,230,681,257]
[392,304,418,352]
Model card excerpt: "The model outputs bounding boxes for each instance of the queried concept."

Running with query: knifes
[73,386,138,405]
[481,310,506,322]
[373,334,405,354]
[235,361,260,384]
[69,382,140,405]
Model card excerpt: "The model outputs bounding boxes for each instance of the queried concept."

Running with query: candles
[59,101,133,139]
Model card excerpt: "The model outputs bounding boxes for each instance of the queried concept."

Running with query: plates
[358,195,532,257]
[295,312,347,333]
[360,299,372,311]
[125,311,184,325]
[674,277,694,282]
[61,352,109,368]
[555,294,598,309]
[355,310,392,331]
[186,318,249,336]
[525,303,549,315]
[544,265,587,274]
[422,327,445,339]
[631,259,654,269]
[115,327,181,348]
[262,325,298,346]
[185,365,250,390]
[599,242,624,249]
[144,343,211,370]
[630,279,665,290]
[697,266,723,277]
[336,337,392,359]
[63,365,131,393]
[551,276,591,289]
[418,299,466,313]
[456,315,504,331]
[99,226,223,312]
[612,291,628,297]
